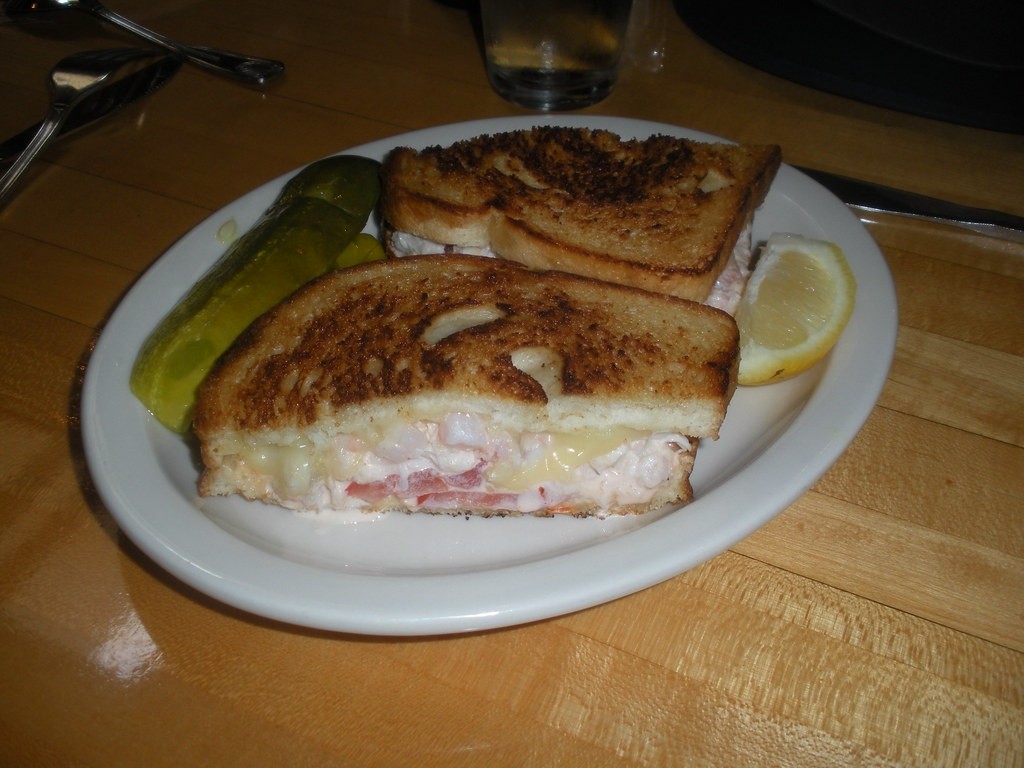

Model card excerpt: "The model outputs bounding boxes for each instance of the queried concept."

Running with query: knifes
[0,53,184,177]
[788,163,1024,242]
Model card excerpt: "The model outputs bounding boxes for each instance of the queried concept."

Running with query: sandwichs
[382,125,782,315]
[195,255,742,518]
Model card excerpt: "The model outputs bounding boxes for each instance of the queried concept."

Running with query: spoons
[0,0,286,84]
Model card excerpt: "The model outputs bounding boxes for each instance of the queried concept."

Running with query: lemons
[735,231,858,385]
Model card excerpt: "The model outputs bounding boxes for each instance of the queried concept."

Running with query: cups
[482,0,634,112]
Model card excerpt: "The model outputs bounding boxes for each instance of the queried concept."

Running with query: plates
[80,116,899,640]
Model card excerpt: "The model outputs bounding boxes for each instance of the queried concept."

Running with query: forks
[0,47,154,202]
